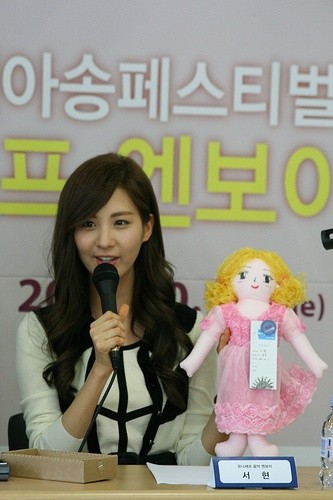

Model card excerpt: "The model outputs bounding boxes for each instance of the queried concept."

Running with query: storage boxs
[0,448,119,484]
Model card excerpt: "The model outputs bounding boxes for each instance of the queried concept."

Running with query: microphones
[92,263,120,371]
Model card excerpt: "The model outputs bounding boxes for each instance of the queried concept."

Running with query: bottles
[319,394,333,490]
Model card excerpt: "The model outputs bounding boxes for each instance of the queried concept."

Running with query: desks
[0,465,333,500]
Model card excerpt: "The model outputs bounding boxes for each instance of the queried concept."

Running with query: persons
[179,248,328,456]
[13,152,231,467]
